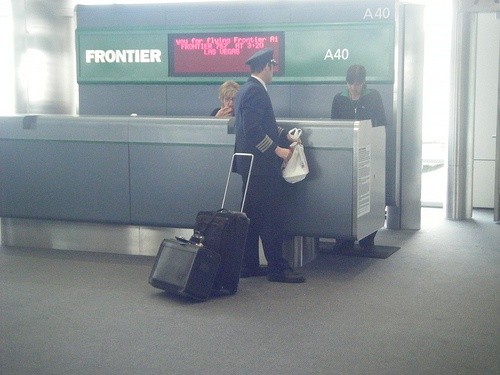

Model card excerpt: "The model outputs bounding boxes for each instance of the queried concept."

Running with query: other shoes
[268,269,305,283]
[240,266,268,277]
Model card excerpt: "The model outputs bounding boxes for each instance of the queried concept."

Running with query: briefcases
[147,232,221,301]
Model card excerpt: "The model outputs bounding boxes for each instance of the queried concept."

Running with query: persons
[232,48,305,283]
[332,65,387,127]
[210,80,239,117]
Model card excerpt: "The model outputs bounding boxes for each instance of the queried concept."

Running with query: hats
[245,48,278,68]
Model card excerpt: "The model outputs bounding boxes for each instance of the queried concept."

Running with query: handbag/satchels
[280,128,309,184]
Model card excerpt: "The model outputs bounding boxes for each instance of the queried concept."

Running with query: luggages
[193,152,254,297]
